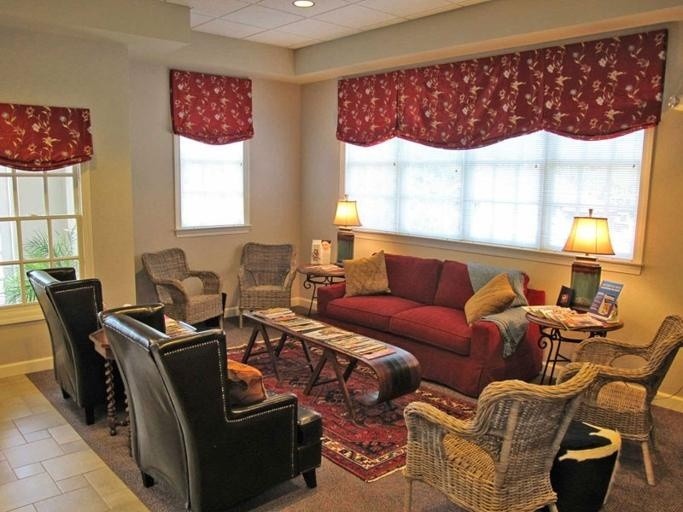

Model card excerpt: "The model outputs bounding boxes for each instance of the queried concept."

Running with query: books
[521,305,603,330]
[324,332,397,360]
[556,285,575,307]
[252,306,312,328]
[310,239,331,265]
[588,280,624,320]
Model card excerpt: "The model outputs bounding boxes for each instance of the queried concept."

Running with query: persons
[321,240,331,264]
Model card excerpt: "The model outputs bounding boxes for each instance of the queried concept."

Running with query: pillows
[342,249,391,298]
[464,271,518,324]
[227,360,268,405]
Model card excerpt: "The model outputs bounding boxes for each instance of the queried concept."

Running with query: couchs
[316,252,546,400]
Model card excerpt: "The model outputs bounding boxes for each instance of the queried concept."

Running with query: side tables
[297,264,346,317]
[549,417,621,512]
[89,323,199,435]
[525,306,623,386]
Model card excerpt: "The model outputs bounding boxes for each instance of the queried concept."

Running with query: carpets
[226,336,478,484]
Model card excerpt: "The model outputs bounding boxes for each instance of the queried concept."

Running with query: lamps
[332,201,363,264]
[562,216,616,306]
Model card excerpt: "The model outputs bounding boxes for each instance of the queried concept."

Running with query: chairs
[26,268,167,426]
[142,248,225,330]
[237,242,297,329]
[96,303,324,511]
[402,360,601,512]
[556,314,683,488]
